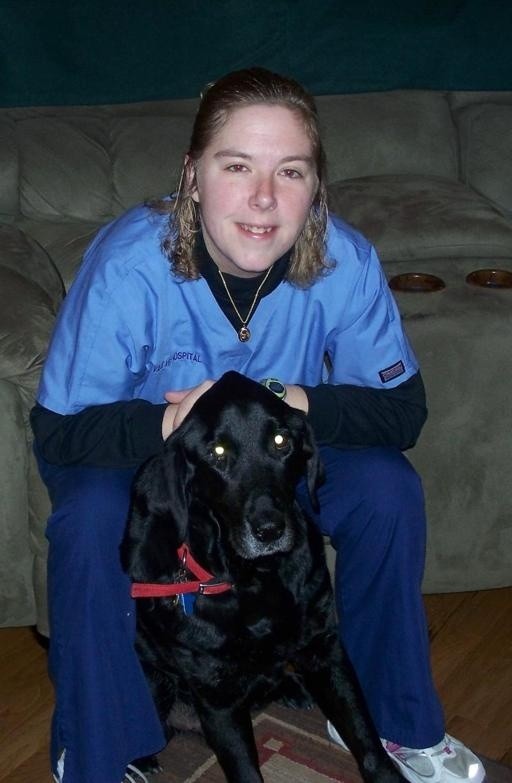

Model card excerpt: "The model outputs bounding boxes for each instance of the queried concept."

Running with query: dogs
[119,368,411,783]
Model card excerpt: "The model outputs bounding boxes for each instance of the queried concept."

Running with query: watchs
[260,376,287,400]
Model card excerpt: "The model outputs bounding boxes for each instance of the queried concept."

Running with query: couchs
[4,84,512,653]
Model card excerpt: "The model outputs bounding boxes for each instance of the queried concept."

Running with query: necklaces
[216,265,274,342]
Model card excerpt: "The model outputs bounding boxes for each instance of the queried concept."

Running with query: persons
[25,64,489,782]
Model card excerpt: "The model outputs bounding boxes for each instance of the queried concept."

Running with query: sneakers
[326,717,486,783]
